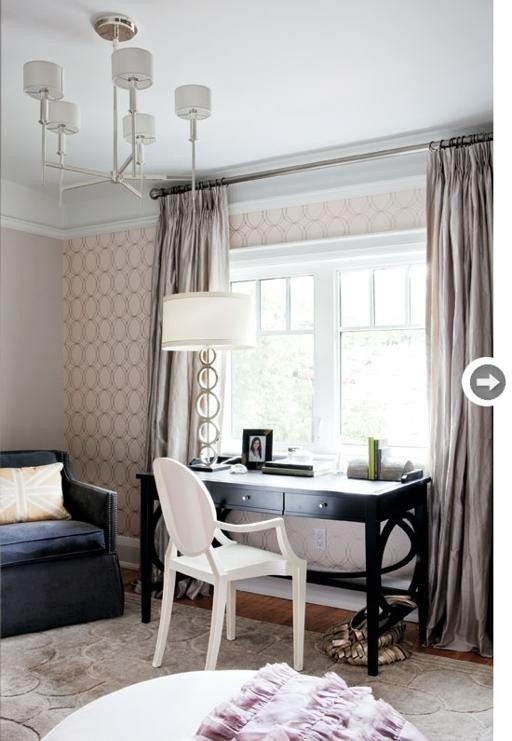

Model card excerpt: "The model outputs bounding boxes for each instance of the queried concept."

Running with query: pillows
[0,463,72,526]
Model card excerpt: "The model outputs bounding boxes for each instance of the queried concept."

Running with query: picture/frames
[241,428,274,471]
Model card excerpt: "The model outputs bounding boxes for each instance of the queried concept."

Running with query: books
[368,436,391,481]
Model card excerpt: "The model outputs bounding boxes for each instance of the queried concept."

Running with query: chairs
[147,457,308,672]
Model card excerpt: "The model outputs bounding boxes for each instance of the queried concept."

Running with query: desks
[136,457,431,677]
[35,671,419,741]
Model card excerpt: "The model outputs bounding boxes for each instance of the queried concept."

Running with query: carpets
[0,591,492,741]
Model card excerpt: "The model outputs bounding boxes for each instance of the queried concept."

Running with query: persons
[248,437,263,461]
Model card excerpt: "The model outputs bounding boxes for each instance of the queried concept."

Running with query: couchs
[0,450,127,639]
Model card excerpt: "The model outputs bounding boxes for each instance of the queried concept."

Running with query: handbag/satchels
[320,595,418,667]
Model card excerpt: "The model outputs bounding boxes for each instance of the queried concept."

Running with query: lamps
[21,10,210,199]
[161,283,255,475]
